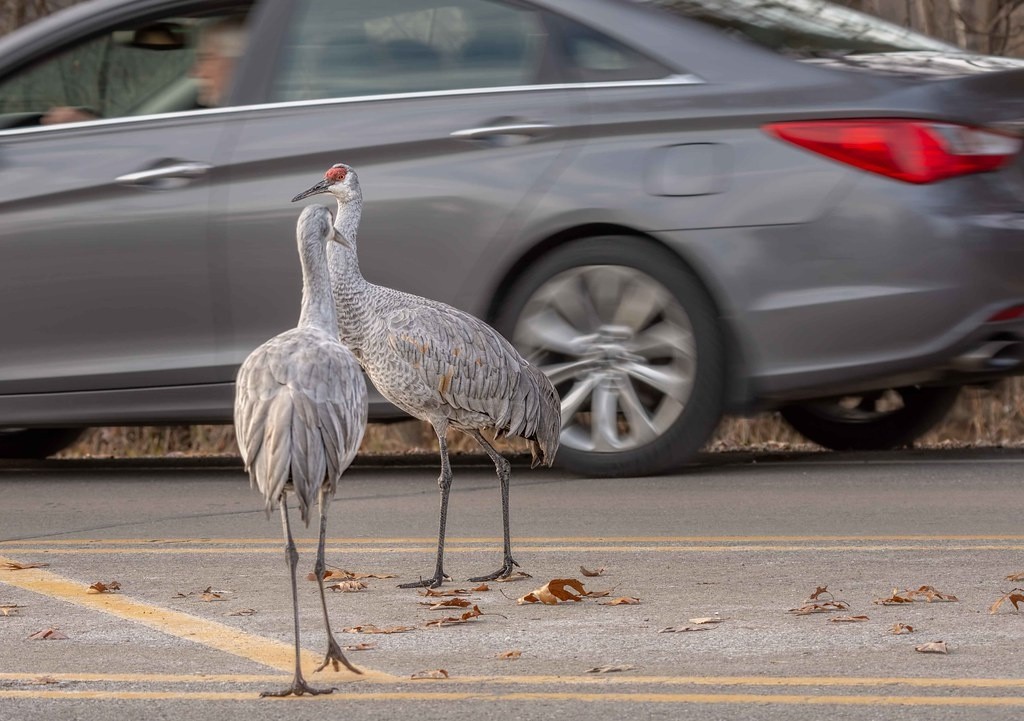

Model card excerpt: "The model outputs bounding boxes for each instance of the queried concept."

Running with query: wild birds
[291,163,562,589]
[233,205,369,696]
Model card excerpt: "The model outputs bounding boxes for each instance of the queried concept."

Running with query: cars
[0,0,1024,477]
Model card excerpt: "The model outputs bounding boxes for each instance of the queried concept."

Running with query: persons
[39,21,241,123]
[141,30,173,44]
